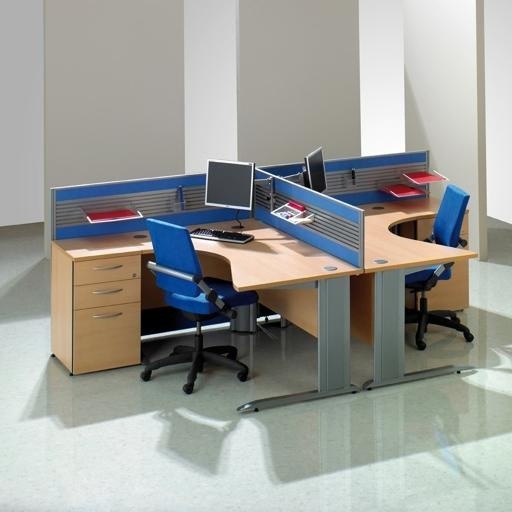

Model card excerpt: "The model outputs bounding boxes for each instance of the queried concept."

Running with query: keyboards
[189,227,255,244]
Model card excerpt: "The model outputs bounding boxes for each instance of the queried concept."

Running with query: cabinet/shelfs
[379,170,450,199]
[397,215,469,310]
[53,244,142,376]
[78,199,143,224]
[270,202,315,227]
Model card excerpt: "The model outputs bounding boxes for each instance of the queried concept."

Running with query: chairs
[405,184,474,352]
[140,218,259,394]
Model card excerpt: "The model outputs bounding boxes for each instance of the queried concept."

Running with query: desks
[52,216,364,414]
[358,196,477,392]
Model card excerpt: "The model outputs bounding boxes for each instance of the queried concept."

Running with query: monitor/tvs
[303,145,328,194]
[205,158,257,212]
